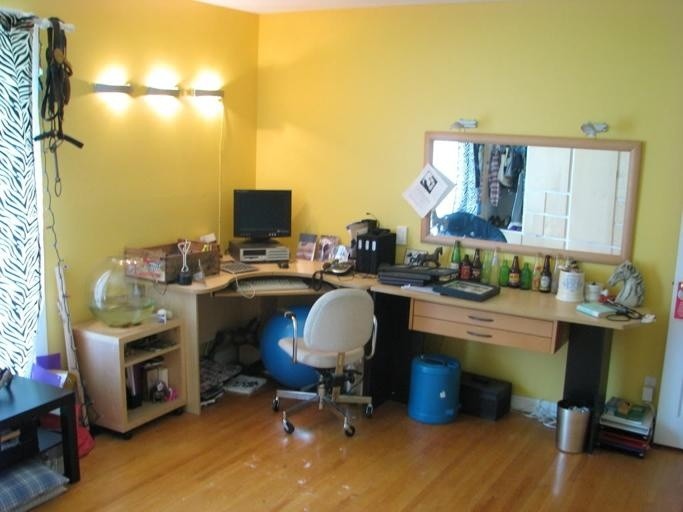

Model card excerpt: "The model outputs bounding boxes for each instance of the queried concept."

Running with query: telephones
[320,259,355,275]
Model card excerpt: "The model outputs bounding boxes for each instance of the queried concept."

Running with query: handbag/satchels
[496,148,512,188]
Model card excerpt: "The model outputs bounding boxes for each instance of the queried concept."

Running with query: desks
[0,375,80,484]
[124,244,651,454]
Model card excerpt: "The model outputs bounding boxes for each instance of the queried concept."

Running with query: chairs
[271,288,378,436]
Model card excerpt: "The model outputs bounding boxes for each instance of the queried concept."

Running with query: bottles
[530,253,544,292]
[550,255,564,294]
[519,263,531,290]
[498,256,510,287]
[460,254,472,280]
[508,256,521,288]
[479,247,492,284]
[489,247,501,287]
[470,248,482,282]
[539,255,551,294]
[449,240,462,280]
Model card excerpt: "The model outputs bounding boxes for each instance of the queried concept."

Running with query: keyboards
[233,277,309,291]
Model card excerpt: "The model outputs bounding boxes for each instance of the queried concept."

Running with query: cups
[556,270,584,303]
[584,281,608,302]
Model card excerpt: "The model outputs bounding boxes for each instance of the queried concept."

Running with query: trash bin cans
[556,400,591,454]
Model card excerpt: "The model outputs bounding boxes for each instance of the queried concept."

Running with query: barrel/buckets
[410,353,458,425]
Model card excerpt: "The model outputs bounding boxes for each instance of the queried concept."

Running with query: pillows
[0,457,70,512]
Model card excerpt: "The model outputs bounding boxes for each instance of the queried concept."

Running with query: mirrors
[420,131,642,266]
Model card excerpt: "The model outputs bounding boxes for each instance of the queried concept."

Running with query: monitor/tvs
[233,189,292,245]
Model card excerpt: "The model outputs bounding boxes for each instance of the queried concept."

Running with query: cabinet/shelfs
[73,316,187,434]
[522,145,572,250]
[566,148,619,254]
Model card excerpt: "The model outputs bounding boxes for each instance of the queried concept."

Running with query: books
[576,302,617,318]
[0,428,21,451]
[596,397,655,459]
[223,374,267,395]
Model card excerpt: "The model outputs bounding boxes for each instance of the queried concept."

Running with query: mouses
[278,260,289,268]
